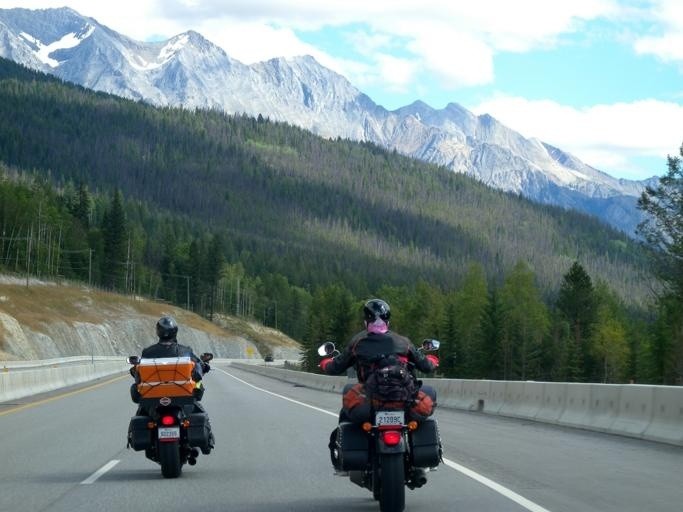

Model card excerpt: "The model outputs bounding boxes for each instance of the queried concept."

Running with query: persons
[129,314,214,455]
[318,298,437,491]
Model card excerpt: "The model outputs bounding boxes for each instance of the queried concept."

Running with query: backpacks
[363,365,416,401]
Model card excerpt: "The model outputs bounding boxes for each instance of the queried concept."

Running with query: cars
[265,354,274,361]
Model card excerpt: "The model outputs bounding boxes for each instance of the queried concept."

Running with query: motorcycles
[122,351,218,477]
[316,338,446,511]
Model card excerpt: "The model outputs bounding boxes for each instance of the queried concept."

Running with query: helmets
[363,299,391,323]
[156,317,177,340]
